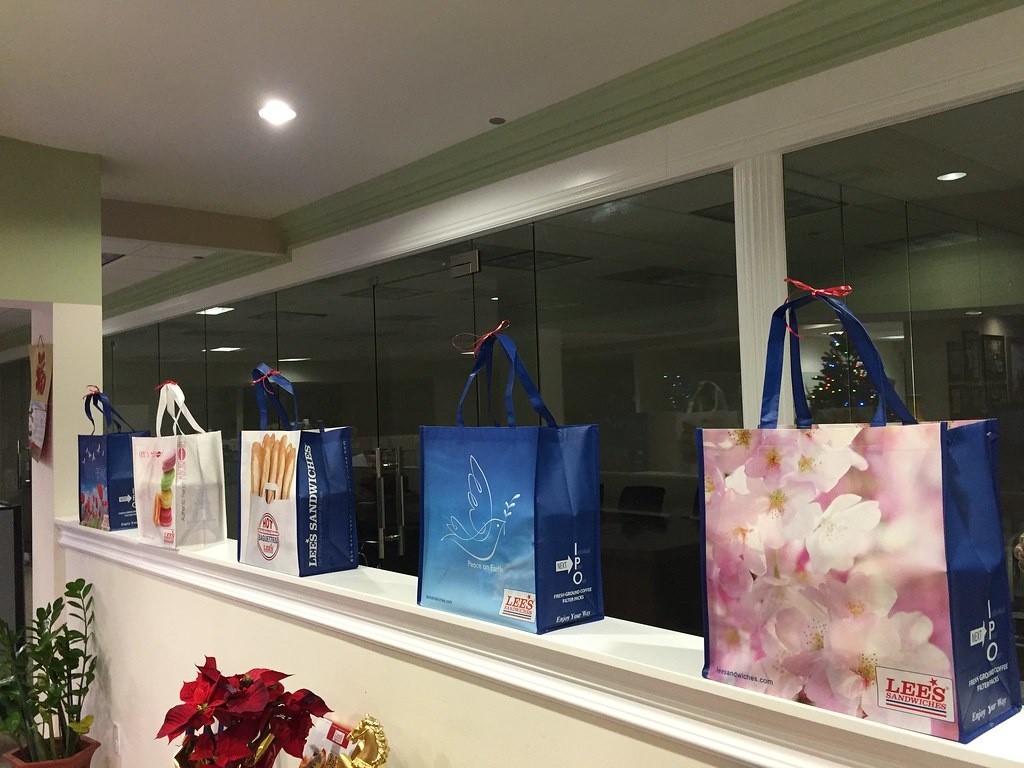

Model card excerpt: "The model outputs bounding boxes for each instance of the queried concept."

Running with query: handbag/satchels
[77,393,151,532]
[415,330,606,636]
[694,294,1024,747]
[130,381,228,550]
[236,362,361,578]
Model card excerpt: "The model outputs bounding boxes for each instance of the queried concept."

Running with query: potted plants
[0,577,101,767]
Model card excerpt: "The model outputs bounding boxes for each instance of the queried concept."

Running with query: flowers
[153,656,333,768]
